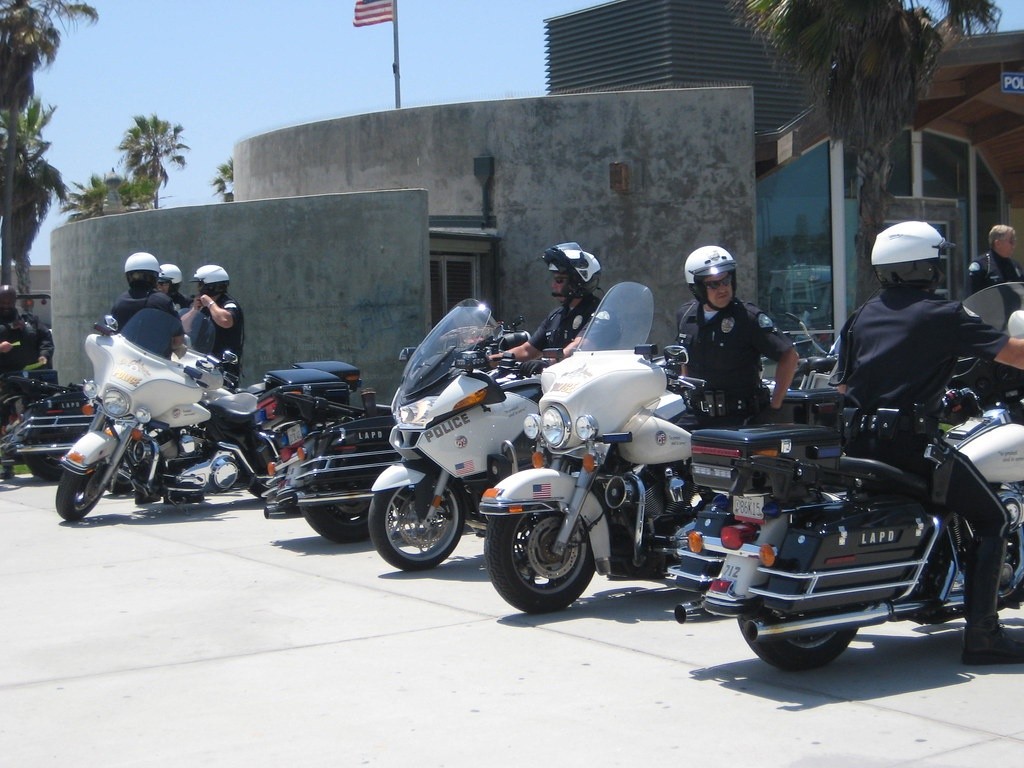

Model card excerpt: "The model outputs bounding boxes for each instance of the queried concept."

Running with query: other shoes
[165,493,205,504]
[135,491,161,504]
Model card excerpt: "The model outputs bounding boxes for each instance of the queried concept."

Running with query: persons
[675,244,799,431]
[111,251,205,505]
[0,282,56,479]
[486,243,621,381]
[970,224,1024,296]
[839,219,1024,665]
[179,263,245,392]
[154,261,194,309]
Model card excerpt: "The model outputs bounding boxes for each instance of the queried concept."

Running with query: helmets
[156,264,183,291]
[683,245,738,302]
[189,265,230,295]
[542,242,601,295]
[871,221,956,287]
[124,251,160,286]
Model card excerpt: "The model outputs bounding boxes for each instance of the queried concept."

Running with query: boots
[962,535,1024,664]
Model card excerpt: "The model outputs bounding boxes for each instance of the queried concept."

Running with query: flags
[354,0,394,27]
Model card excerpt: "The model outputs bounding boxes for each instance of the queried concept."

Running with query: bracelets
[208,300,215,309]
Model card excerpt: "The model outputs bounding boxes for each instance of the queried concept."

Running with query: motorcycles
[0,315,366,523]
[257,280,1024,674]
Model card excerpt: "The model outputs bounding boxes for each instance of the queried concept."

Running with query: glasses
[703,274,732,290]
[553,276,568,285]
